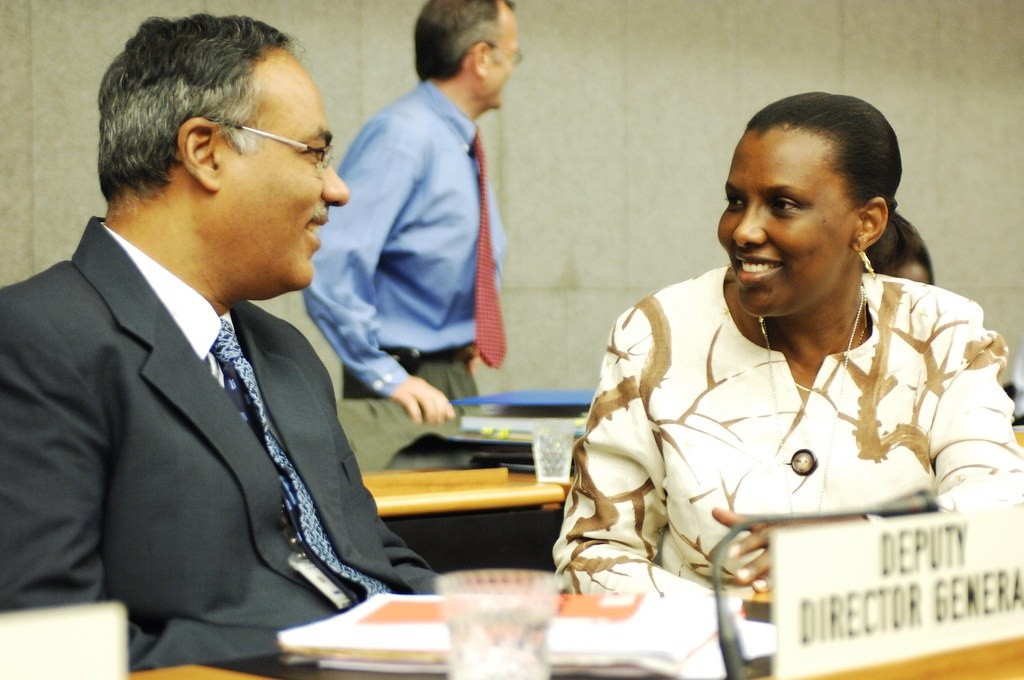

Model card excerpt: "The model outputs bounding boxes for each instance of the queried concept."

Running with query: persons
[303,0,525,467]
[553,91,1022,611]
[1,16,441,672]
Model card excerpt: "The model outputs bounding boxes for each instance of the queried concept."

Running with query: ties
[209,319,393,603]
[473,127,507,370]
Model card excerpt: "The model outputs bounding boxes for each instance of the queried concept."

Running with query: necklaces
[759,288,870,514]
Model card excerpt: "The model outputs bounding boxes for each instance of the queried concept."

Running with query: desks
[362,465,579,518]
[131,576,1024,680]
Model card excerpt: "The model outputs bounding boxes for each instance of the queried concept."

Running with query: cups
[436,567,559,680]
[532,420,573,484]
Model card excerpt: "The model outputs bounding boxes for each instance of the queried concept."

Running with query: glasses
[187,116,333,174]
[479,40,524,65]
